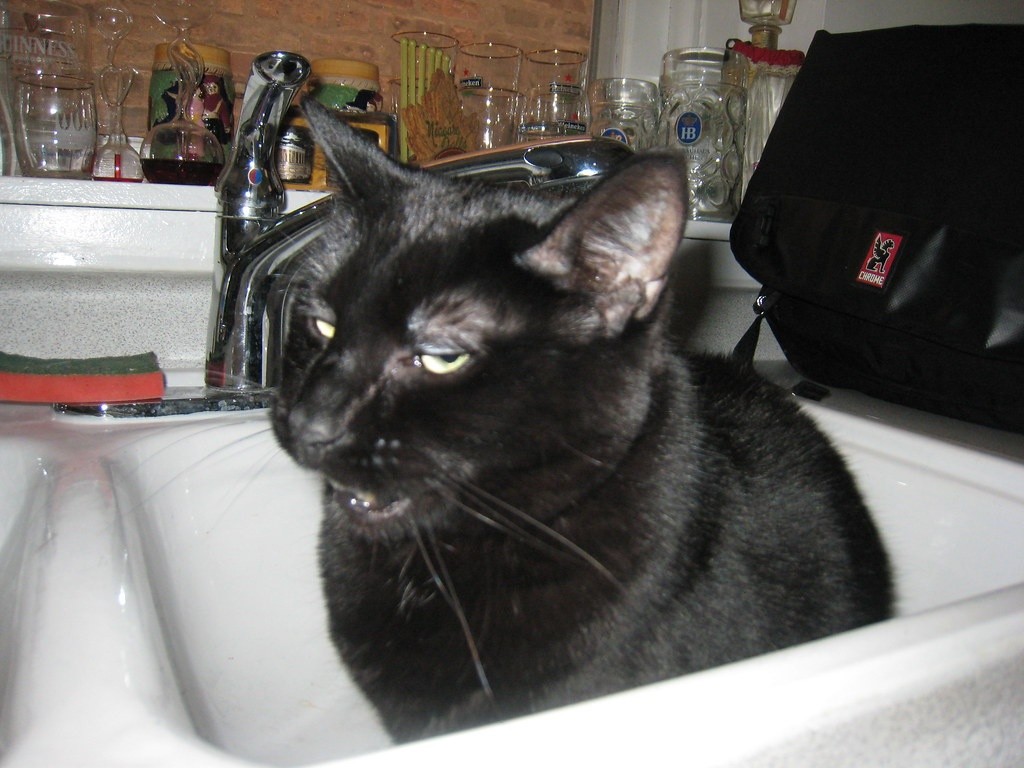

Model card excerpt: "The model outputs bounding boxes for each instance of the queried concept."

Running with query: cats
[268,91,900,745]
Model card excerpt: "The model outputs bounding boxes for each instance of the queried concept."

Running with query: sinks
[1,366,1024,767]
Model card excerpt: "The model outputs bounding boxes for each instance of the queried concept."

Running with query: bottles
[305,58,381,190]
[0,0,234,183]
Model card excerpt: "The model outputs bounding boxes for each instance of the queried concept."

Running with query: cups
[388,31,749,222]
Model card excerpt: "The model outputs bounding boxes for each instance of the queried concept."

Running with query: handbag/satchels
[730,24,1024,433]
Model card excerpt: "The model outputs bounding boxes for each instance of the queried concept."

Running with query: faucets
[51,49,636,419]
